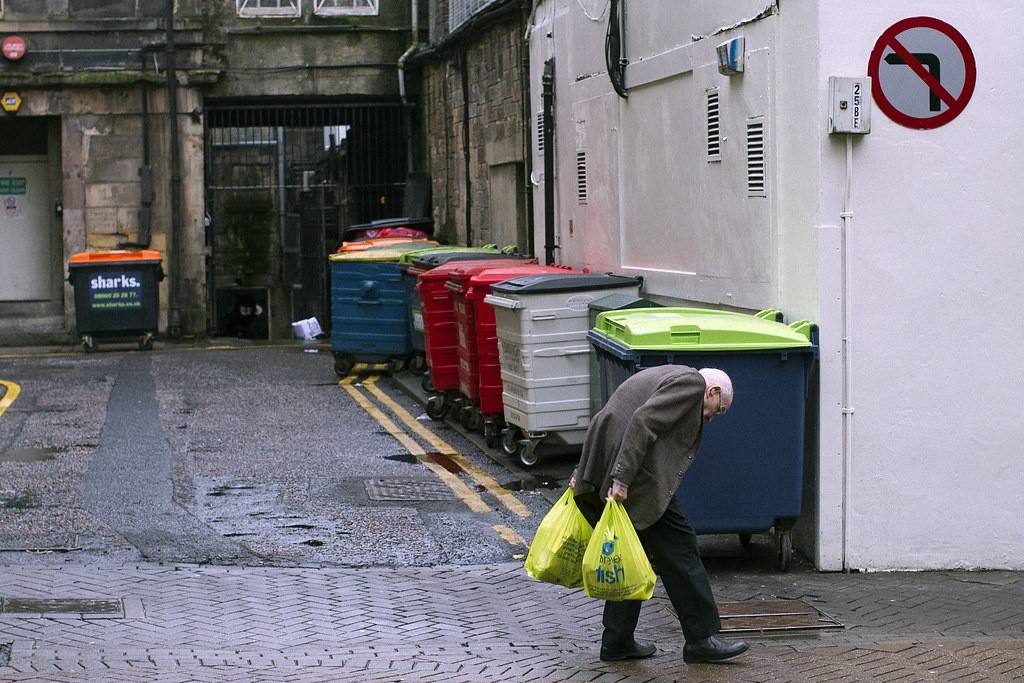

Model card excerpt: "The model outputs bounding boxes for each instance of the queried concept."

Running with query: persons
[567,365,750,663]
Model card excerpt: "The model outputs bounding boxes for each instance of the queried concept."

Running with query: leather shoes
[600,640,657,661]
[682,635,750,663]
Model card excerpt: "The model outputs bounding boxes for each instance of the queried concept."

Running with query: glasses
[713,389,725,415]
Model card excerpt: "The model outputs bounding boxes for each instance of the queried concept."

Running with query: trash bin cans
[583,306,819,571]
[483,270,645,466]
[328,215,589,450]
[64,248,166,353]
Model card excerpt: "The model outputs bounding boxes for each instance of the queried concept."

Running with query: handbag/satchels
[524,486,596,589]
[582,494,658,601]
